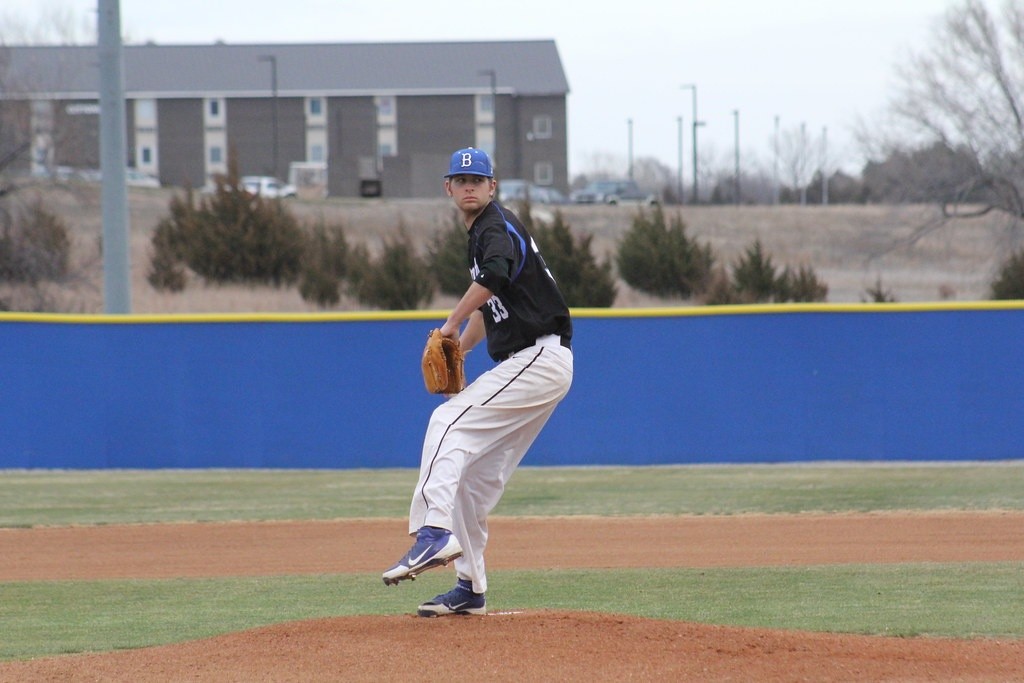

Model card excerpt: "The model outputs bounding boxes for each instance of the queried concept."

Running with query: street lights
[477,67,499,179]
[680,83,698,203]
[692,121,705,202]
[257,53,279,176]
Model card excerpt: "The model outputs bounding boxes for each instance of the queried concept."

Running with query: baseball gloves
[421,327,464,394]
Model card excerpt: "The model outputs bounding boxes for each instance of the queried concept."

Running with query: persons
[382,145,574,617]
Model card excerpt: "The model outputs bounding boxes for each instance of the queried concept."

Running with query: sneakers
[417,577,487,618]
[382,526,464,586]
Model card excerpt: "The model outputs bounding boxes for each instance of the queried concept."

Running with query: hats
[444,147,492,179]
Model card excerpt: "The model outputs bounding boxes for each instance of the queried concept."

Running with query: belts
[503,336,572,360]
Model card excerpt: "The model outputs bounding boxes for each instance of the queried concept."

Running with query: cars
[238,175,298,200]
[497,179,640,206]
[97,167,161,190]
